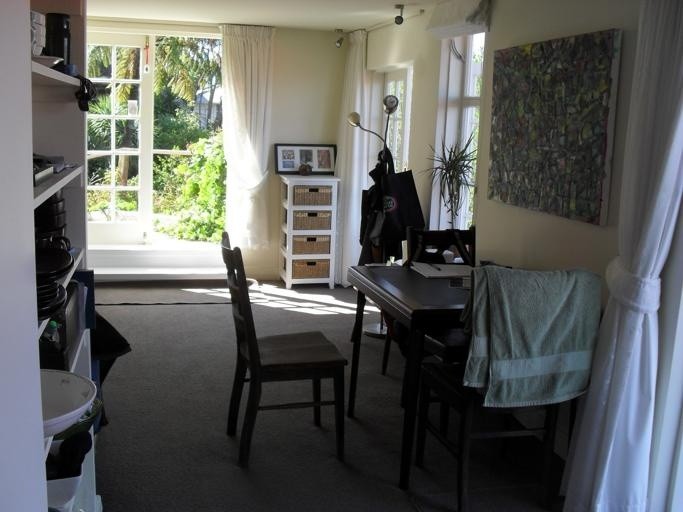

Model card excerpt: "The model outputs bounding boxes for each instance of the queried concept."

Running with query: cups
[38,191,71,249]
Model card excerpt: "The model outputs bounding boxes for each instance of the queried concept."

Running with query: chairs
[381,226,477,408]
[414,267,602,512]
[221,231,348,469]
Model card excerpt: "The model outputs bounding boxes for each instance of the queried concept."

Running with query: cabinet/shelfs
[0,0,104,512]
[278,174,341,290]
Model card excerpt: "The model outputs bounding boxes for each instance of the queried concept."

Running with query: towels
[460,264,602,408]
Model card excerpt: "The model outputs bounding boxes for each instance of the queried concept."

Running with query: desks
[347,266,467,492]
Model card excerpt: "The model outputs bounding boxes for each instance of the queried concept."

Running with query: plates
[35,55,63,68]
[36,245,76,316]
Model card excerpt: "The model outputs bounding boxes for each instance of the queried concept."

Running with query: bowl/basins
[41,367,98,440]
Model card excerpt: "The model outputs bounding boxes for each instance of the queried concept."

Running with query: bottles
[44,13,71,63]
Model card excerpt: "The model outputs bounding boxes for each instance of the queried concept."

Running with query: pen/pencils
[428,263,441,270]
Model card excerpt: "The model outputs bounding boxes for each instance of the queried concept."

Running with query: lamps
[395,4,404,25]
[334,29,344,48]
[346,95,398,337]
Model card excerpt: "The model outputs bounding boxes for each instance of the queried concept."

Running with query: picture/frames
[274,143,337,175]
